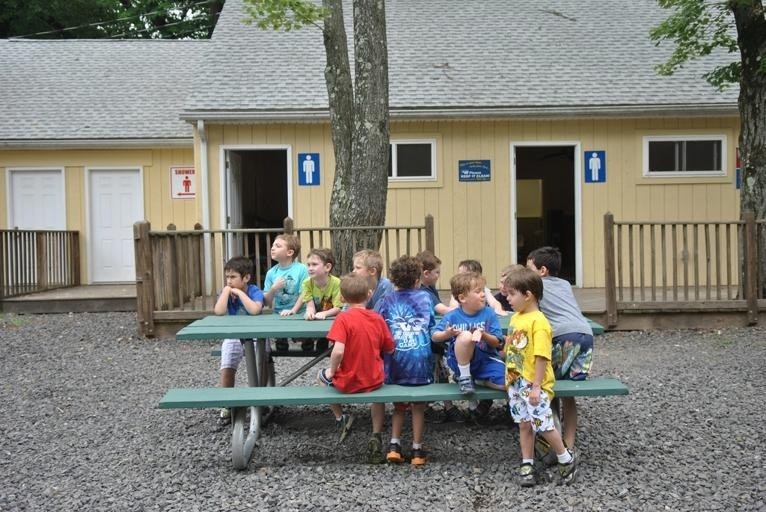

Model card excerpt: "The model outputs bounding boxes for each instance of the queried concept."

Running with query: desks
[171,309,607,471]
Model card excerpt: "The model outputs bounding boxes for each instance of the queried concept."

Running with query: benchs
[157,375,630,475]
[203,343,337,388]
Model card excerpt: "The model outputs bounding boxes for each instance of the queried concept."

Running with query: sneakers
[216,408,231,426]
[443,405,467,423]
[518,462,540,487]
[456,374,476,395]
[367,433,383,465]
[475,398,493,416]
[335,414,356,444]
[557,447,577,486]
[411,448,429,466]
[386,442,406,463]
[423,407,445,423]
[563,438,581,466]
[535,434,556,465]
